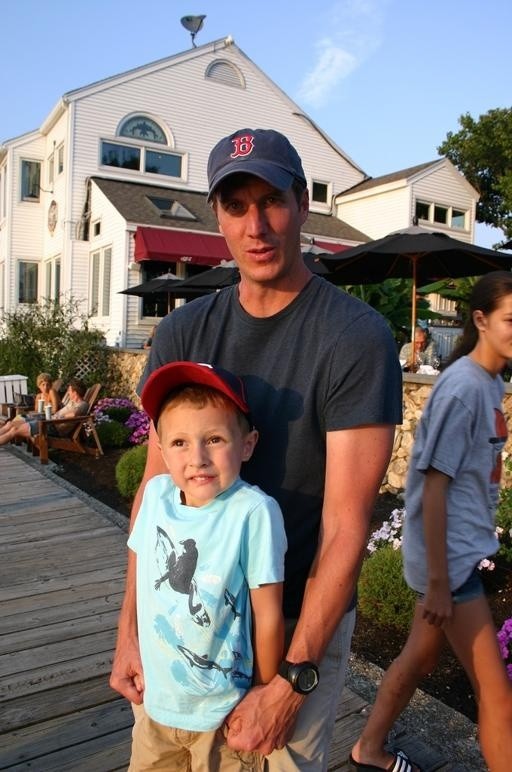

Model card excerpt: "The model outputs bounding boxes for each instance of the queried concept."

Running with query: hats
[206,128,307,205]
[140,360,255,435]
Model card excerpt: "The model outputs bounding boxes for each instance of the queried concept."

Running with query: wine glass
[415,349,439,376]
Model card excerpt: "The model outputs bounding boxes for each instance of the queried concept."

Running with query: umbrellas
[317,216,512,375]
[117,268,215,314]
[174,241,334,288]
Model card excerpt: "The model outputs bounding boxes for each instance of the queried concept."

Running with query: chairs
[0,379,64,446]
[26,383,103,464]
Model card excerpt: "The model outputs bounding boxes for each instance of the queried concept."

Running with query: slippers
[348,747,424,772]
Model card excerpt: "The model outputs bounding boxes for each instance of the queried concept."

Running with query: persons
[398,326,441,377]
[109,127,402,772]
[0,381,88,445]
[351,270,512,772]
[34,373,61,412]
[126,361,289,771]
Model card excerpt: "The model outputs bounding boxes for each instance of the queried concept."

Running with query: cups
[45,406,51,420]
[38,400,45,412]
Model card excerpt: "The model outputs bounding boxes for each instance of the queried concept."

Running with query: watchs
[277,659,320,694]
